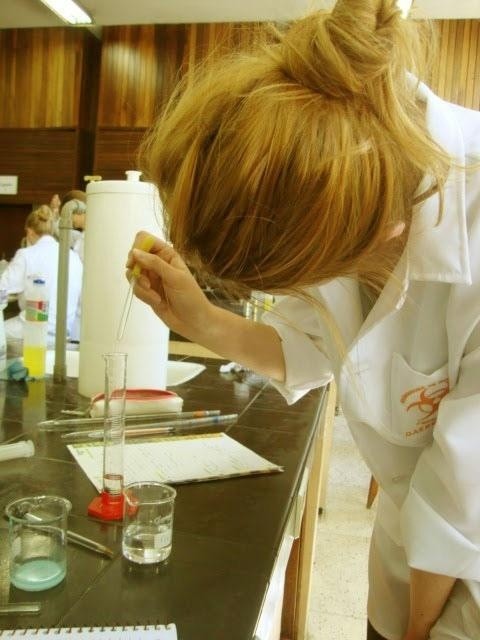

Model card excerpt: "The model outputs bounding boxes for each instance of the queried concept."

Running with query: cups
[123,482,176,563]
[5,496,73,591]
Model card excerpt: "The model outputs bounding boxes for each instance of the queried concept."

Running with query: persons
[126,0,480,640]
[0,190,87,344]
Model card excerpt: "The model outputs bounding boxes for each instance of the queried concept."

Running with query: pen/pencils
[16,509,114,556]
[37,410,238,438]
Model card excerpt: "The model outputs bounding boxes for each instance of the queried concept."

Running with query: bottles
[22,274,49,379]
[104,352,128,495]
